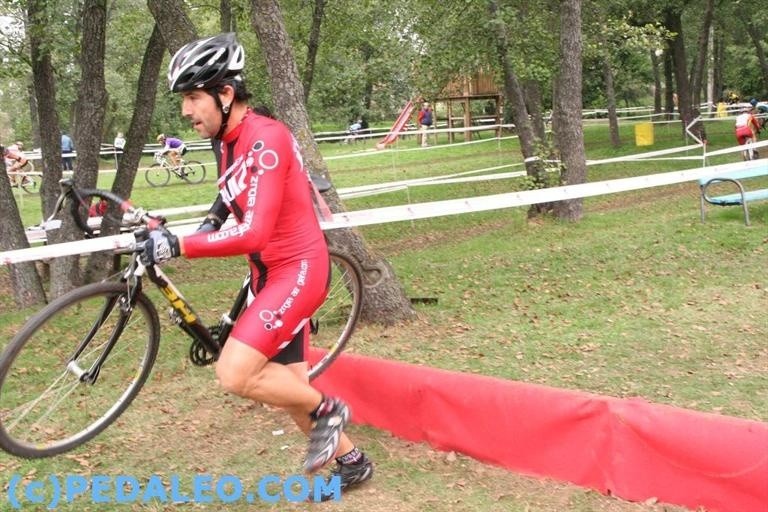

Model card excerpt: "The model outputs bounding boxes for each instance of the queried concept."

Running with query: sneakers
[171,166,188,177]
[302,394,351,472]
[10,179,33,188]
[306,455,374,500]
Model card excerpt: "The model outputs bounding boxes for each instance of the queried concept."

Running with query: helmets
[168,30,247,94]
[155,133,166,142]
[728,91,756,114]
[16,141,24,147]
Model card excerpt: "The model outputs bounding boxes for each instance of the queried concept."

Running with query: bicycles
[340,129,365,146]
[6,157,42,194]
[0,177,364,458]
[145,151,206,187]
[744,112,768,161]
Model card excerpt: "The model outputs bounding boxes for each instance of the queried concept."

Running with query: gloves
[192,217,221,235]
[113,230,181,267]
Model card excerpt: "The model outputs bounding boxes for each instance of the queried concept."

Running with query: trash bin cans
[634,121,655,146]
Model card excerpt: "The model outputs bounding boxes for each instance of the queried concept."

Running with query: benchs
[699,164,768,226]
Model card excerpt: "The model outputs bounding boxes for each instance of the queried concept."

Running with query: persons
[5,140,25,167]
[134,31,373,503]
[729,99,737,115]
[344,114,371,145]
[59,128,75,171]
[113,129,126,164]
[749,98,767,134]
[417,101,432,147]
[0,143,37,189]
[734,106,760,161]
[155,132,188,171]
[714,97,728,117]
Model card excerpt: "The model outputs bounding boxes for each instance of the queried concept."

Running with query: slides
[375,94,422,148]
